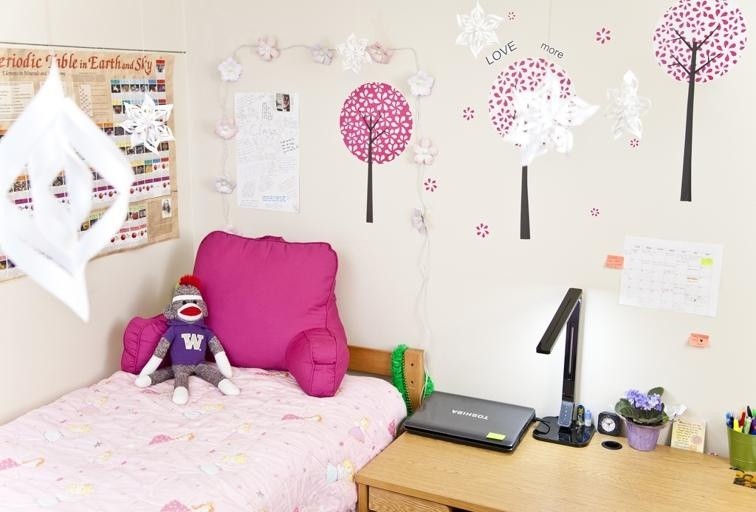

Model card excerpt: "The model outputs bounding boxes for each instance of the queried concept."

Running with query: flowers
[614,387,669,425]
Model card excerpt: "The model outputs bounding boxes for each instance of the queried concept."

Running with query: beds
[0,345,425,512]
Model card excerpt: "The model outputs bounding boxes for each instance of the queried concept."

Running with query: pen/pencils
[725,405,756,435]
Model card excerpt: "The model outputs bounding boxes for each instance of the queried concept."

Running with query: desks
[353,420,756,512]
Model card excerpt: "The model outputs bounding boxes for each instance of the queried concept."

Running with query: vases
[626,420,665,452]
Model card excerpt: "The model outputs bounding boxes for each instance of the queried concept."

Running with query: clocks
[598,412,621,436]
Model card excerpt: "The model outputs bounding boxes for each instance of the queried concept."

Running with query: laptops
[404,390,535,453]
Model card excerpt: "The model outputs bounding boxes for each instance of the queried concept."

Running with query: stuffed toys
[132,271,243,405]
